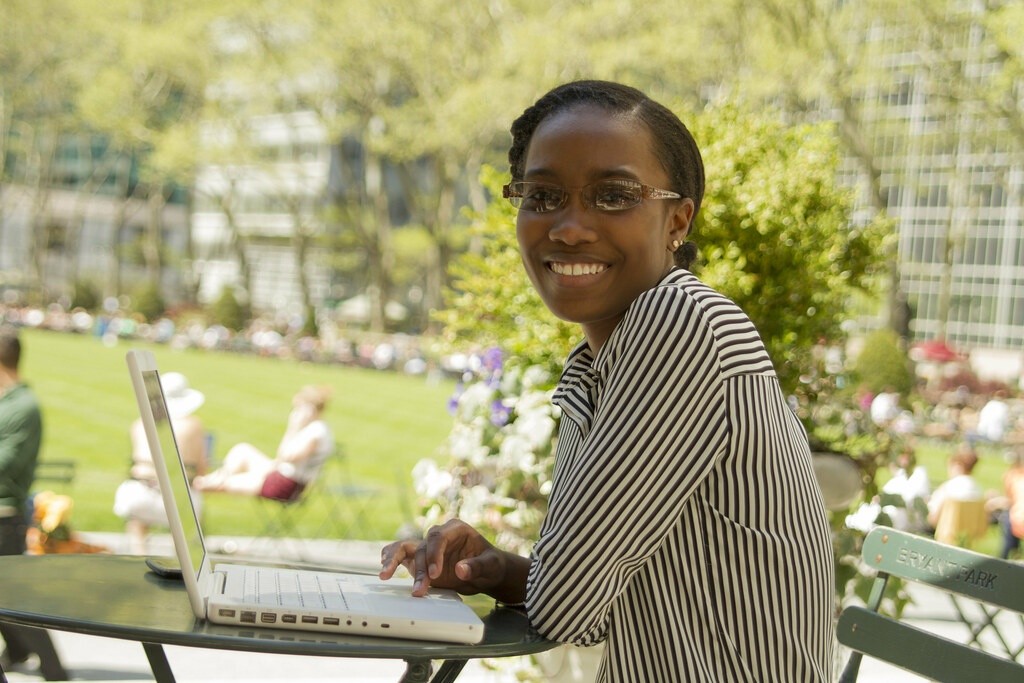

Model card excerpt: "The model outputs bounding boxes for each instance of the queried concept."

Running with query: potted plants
[427,90,929,683]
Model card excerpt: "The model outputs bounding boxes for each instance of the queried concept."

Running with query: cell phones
[145,557,185,578]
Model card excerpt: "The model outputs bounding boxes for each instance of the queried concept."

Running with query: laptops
[123,348,484,643]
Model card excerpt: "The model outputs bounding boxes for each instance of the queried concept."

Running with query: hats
[160,372,203,417]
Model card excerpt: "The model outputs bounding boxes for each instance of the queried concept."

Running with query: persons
[853,380,1019,444]
[807,333,845,379]
[0,334,45,673]
[194,378,334,507]
[25,490,107,555]
[928,443,984,534]
[376,78,838,683]
[987,449,1024,559]
[884,447,928,528]
[111,371,208,556]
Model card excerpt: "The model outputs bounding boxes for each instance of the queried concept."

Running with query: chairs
[248,445,345,558]
[934,501,991,548]
[837,525,1024,682]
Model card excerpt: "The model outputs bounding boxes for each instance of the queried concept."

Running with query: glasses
[503,177,682,213]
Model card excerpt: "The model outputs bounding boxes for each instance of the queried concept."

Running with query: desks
[0,555,557,683]
